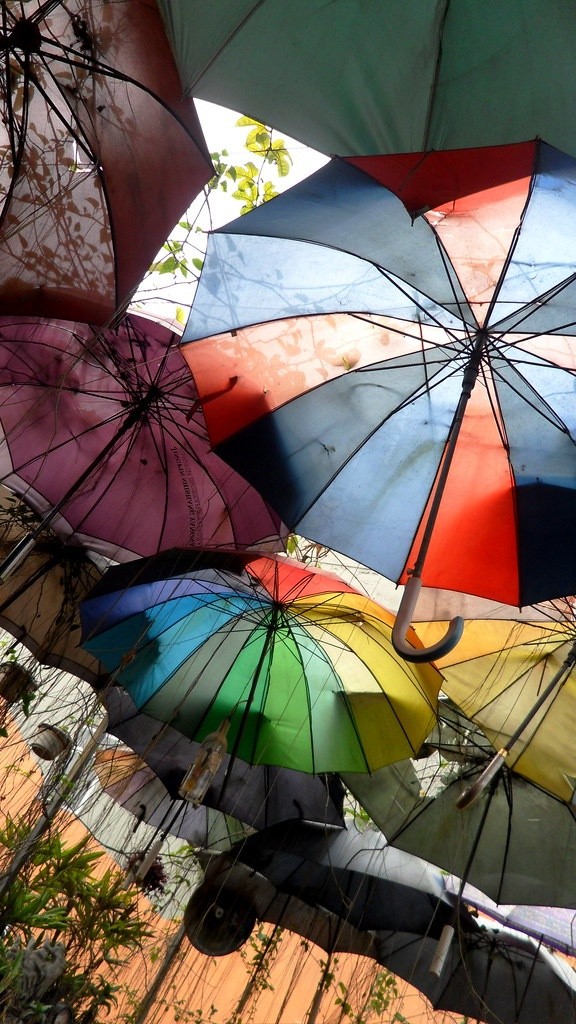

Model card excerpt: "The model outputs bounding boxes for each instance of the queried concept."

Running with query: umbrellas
[0,0,576,1024]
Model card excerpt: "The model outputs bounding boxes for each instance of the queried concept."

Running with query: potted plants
[32,721,72,760]
[0,654,36,717]
